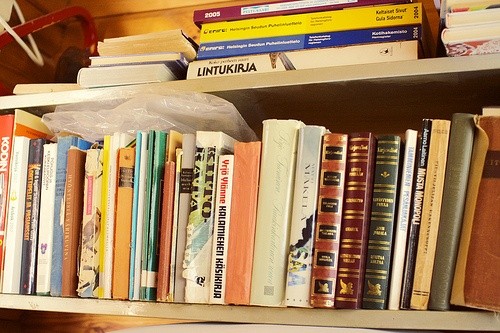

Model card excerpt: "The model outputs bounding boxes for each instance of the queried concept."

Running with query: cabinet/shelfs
[0,0,500,333]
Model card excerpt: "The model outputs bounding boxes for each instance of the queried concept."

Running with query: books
[0,108,500,313]
[77,29,199,88]
[13,84,80,94]
[441,0,500,57]
[187,0,431,80]
